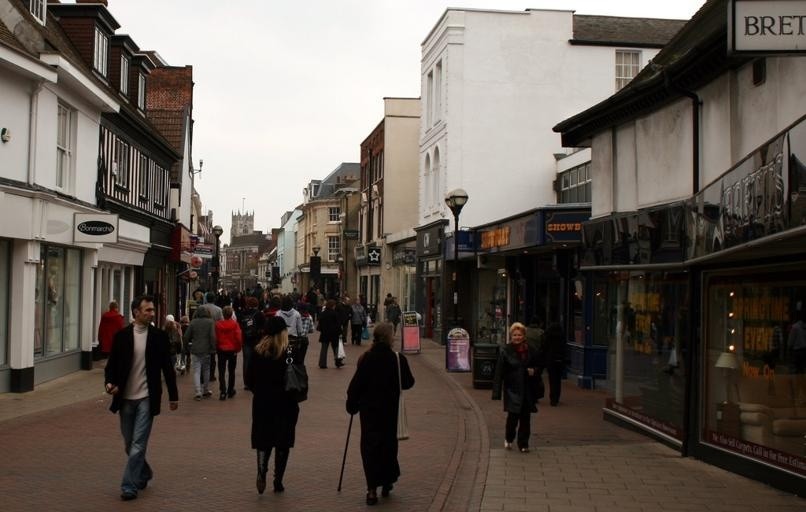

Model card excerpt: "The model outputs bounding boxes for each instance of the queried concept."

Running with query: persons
[104,295,178,500]
[490,322,538,453]
[162,287,366,401]
[789,301,806,381]
[244,315,308,495]
[526,316,567,406]
[345,321,416,506]
[384,293,401,335]
[98,301,127,360]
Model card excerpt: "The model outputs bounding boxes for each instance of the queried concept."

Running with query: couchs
[730,374,805,457]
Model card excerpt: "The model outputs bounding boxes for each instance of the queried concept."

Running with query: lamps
[714,353,739,404]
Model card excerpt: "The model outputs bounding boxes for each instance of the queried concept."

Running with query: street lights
[309,246,321,289]
[443,188,470,324]
[212,225,224,292]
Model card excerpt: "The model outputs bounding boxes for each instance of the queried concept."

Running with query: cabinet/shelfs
[717,404,740,443]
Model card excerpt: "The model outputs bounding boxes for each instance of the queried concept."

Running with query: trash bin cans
[366,304,377,323]
[473,344,501,390]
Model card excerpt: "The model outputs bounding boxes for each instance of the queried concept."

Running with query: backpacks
[243,311,258,329]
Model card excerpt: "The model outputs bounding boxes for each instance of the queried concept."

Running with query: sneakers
[195,389,236,401]
[505,440,512,448]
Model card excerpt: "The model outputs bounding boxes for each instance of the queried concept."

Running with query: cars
[219,278,236,287]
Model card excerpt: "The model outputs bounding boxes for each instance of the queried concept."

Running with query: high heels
[272,479,284,492]
[367,492,377,505]
[256,468,268,494]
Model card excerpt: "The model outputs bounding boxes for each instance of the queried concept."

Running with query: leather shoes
[121,491,137,500]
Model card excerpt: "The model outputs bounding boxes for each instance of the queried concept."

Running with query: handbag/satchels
[284,345,308,402]
[396,392,409,440]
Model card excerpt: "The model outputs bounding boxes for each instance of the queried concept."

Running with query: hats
[268,316,290,332]
[166,315,174,321]
